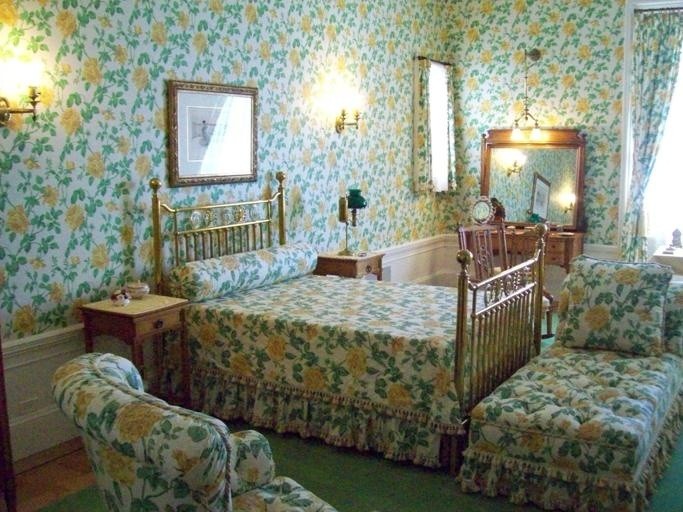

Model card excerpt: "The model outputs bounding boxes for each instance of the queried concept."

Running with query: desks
[457,227,583,276]
[653,244,682,273]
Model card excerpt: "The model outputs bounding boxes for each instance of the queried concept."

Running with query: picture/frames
[167,79,257,187]
[529,172,550,220]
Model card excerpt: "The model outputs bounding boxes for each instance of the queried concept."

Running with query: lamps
[339,189,367,256]
[0,60,42,126]
[336,92,365,132]
[510,49,543,143]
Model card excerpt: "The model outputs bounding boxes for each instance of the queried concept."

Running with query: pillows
[167,242,318,303]
[555,256,672,355]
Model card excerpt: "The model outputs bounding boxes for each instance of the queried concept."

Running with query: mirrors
[470,195,494,223]
[490,197,505,221]
[479,128,586,231]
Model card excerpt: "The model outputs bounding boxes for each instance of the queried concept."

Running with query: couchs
[50,351,341,512]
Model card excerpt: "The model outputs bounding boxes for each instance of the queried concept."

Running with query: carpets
[41,312,682,512]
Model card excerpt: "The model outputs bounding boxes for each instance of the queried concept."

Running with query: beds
[149,172,547,478]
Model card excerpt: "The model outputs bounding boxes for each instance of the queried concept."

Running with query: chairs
[457,222,508,288]
[510,227,555,339]
[456,279,682,512]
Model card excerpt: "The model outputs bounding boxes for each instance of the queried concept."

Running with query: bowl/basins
[127,283,150,297]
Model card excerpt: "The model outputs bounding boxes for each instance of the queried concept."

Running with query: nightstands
[77,295,190,408]
[317,251,385,281]
